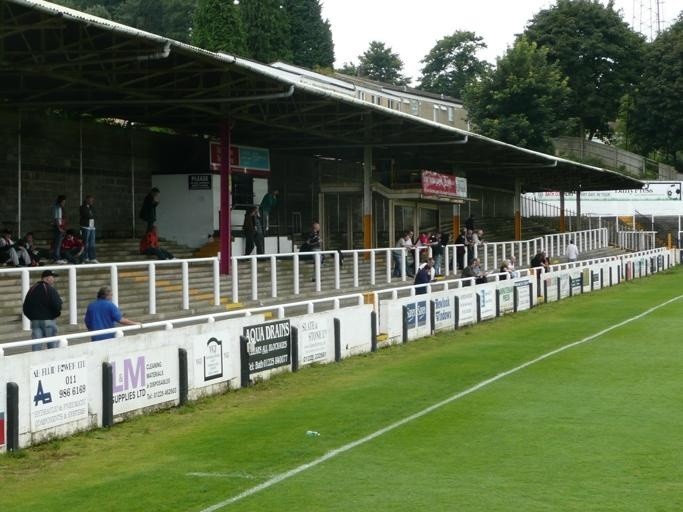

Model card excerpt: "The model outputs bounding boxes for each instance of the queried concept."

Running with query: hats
[41,270,59,279]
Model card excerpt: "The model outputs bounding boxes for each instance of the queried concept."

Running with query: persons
[459,259,487,287]
[415,257,439,284]
[530,253,548,270]
[472,256,488,284]
[242,208,257,263]
[390,225,489,278]
[249,206,266,261]
[565,239,579,268]
[464,213,474,231]
[304,220,325,282]
[139,186,160,233]
[540,250,551,270]
[508,255,518,273]
[84,285,143,343]
[413,263,432,295]
[139,219,180,260]
[0,193,99,268]
[257,186,279,236]
[22,270,64,350]
[499,259,515,281]
